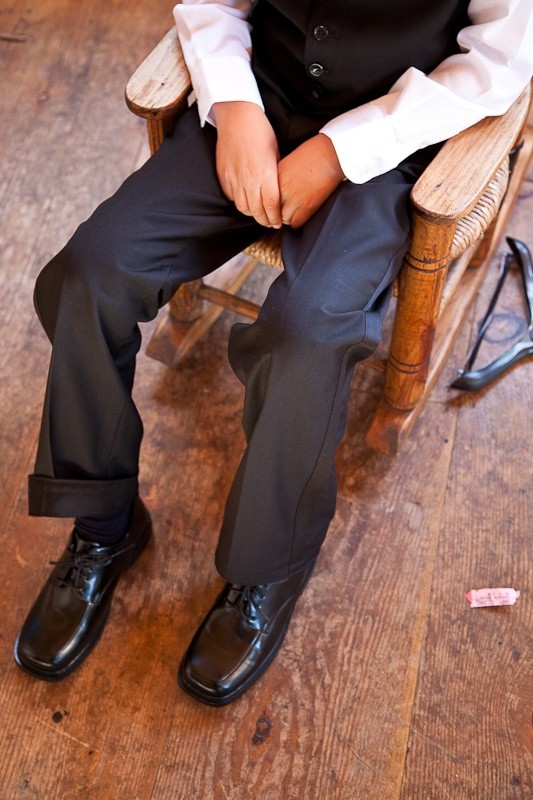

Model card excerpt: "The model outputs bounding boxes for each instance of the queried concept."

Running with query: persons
[14,0,533,707]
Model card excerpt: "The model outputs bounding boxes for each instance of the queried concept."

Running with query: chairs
[122,25,532,454]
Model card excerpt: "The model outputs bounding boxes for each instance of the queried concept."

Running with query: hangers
[453,236,533,388]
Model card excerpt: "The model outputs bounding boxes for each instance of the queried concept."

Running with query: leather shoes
[177,558,316,707]
[14,496,152,682]
